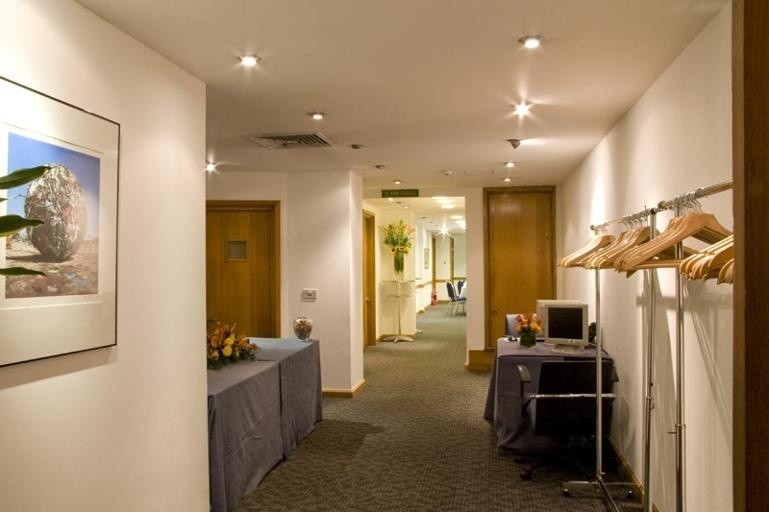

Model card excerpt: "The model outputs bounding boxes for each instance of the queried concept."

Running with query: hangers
[550,185,736,287]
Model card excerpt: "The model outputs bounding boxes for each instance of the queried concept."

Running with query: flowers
[511,313,543,349]
[379,217,418,282]
[206,316,262,372]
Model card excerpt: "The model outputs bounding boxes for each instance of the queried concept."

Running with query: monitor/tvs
[543,301,589,354]
[537,299,546,329]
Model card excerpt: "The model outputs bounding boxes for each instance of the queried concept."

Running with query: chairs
[445,276,467,316]
[510,356,622,486]
[504,313,526,338]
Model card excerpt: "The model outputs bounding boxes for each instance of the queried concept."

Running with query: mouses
[509,335,516,341]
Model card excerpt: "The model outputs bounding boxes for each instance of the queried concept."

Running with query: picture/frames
[0,74,121,369]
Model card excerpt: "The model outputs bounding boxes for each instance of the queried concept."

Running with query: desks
[206,357,286,511]
[481,333,613,460]
[237,332,323,460]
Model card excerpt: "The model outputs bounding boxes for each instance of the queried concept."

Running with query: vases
[518,331,537,349]
[393,250,405,281]
[293,315,313,342]
